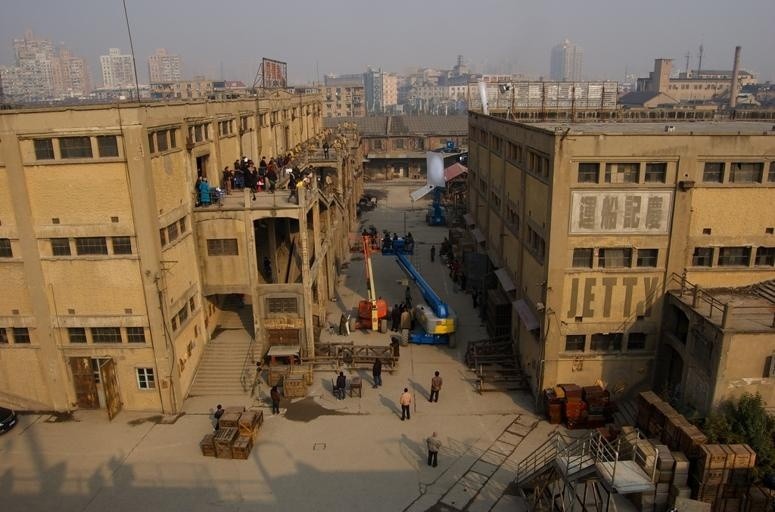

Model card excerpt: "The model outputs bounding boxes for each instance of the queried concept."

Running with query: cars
[0,406,17,435]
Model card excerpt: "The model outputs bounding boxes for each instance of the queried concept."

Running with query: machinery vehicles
[426,186,448,227]
[348,232,389,334]
[381,236,459,349]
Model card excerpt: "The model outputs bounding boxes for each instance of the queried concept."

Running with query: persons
[407,232,414,251]
[438,236,450,257]
[399,387,413,421]
[269,384,281,415]
[398,303,405,320]
[393,232,398,240]
[430,245,436,262]
[337,371,347,401]
[322,141,330,160]
[212,404,225,432]
[369,225,377,244]
[361,228,369,236]
[426,430,441,467]
[391,304,401,332]
[372,357,383,388]
[382,234,392,249]
[194,149,336,210]
[427,370,443,403]
